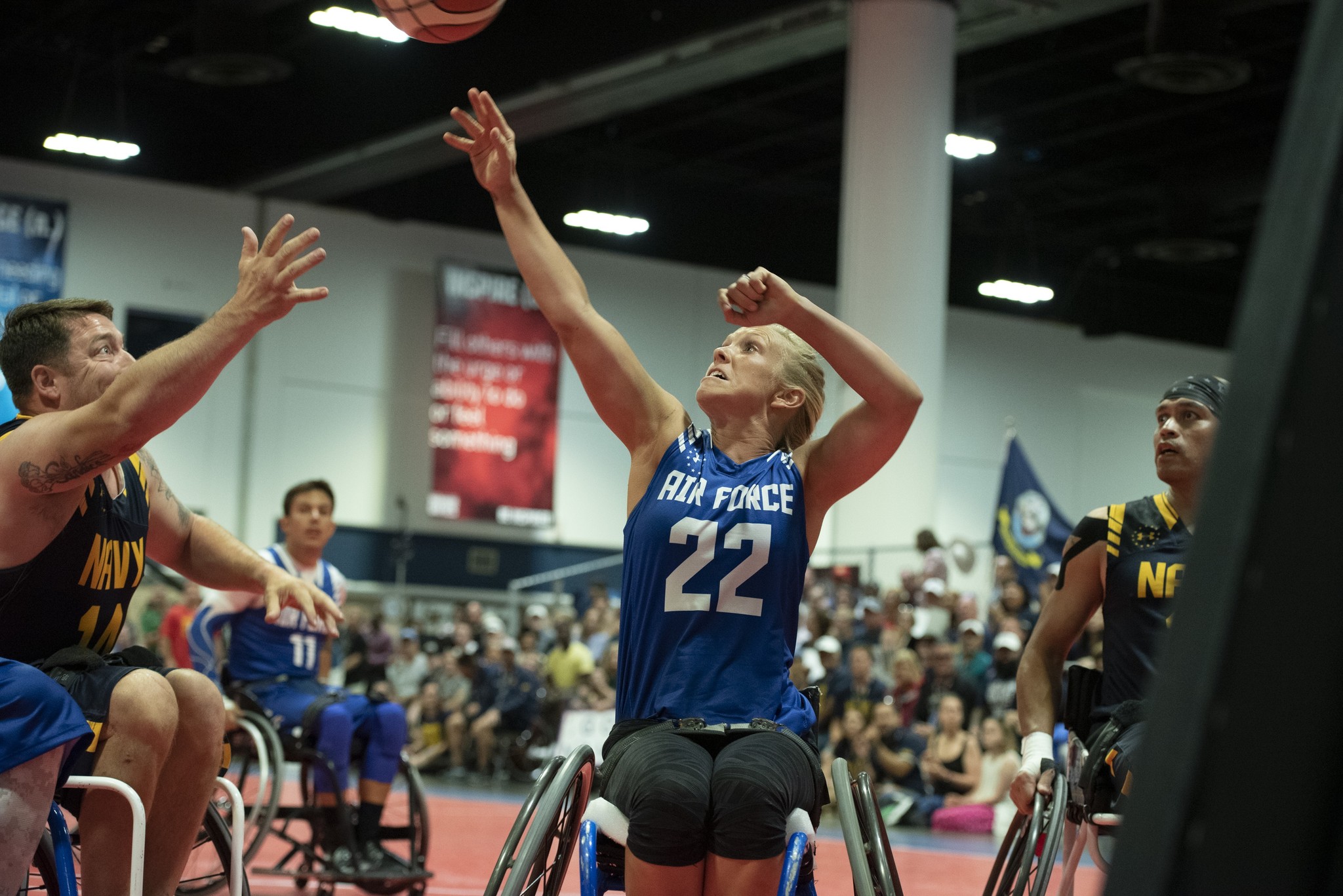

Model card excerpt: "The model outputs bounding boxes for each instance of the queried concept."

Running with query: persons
[1009,374,1231,837]
[0,657,95,896]
[915,530,947,586]
[329,529,1104,831]
[108,578,226,673]
[184,478,409,874]
[442,87,925,896]
[0,211,329,896]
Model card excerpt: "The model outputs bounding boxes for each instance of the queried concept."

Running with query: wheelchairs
[981,661,1123,896]
[17,775,250,896]
[482,680,905,896]
[173,656,431,896]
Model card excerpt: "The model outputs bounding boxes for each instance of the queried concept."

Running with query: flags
[989,437,1077,586]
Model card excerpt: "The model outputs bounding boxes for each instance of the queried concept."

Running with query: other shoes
[324,839,408,880]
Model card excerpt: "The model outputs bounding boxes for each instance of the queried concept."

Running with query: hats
[500,636,519,653]
[482,617,506,635]
[958,619,986,637]
[524,603,549,624]
[813,635,842,653]
[921,577,946,597]
[992,631,1022,652]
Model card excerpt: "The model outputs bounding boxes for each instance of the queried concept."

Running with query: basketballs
[370,0,505,44]
